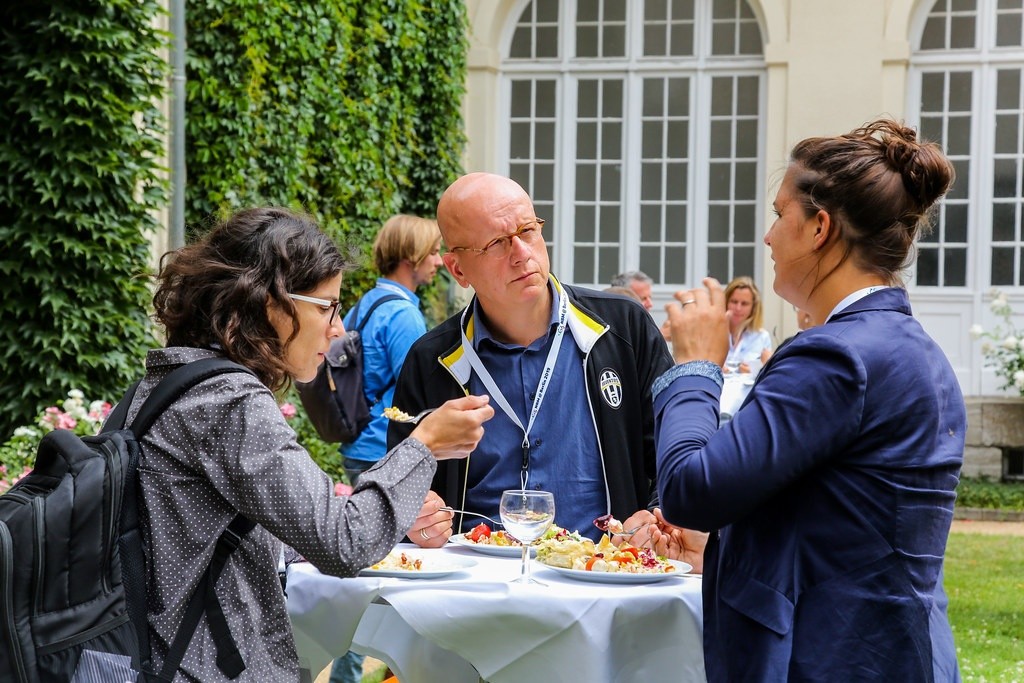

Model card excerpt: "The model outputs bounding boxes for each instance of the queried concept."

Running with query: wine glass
[500,489,554,586]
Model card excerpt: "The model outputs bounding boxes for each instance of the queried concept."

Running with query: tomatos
[586,557,601,571]
[622,548,638,560]
[614,553,631,563]
[472,524,491,541]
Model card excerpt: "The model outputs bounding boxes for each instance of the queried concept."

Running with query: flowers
[966,287,1024,395]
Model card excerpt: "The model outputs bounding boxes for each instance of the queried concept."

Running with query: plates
[360,549,478,578]
[448,531,538,559]
[535,556,693,583]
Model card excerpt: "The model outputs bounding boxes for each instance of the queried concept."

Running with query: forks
[391,408,438,423]
[613,523,660,536]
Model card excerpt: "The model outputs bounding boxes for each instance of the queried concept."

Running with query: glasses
[451,215,545,260]
[287,294,343,325]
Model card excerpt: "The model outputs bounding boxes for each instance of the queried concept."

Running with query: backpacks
[0,357,260,683]
[294,294,409,443]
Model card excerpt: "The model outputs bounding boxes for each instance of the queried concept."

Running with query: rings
[420,529,429,540]
[682,300,696,306]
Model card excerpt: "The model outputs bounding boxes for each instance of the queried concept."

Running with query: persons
[601,272,671,342]
[342,215,443,489]
[385,172,675,552]
[722,276,772,374]
[100,207,495,683]
[650,120,967,683]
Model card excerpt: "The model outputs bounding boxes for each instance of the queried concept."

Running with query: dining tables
[282,542,710,683]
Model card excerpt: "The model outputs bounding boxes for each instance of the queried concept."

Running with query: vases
[964,399,1024,481]
